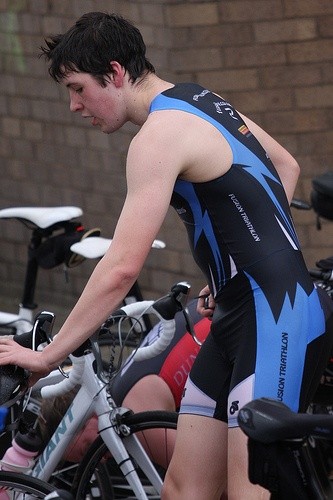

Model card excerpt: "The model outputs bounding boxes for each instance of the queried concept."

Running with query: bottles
[0,428,41,500]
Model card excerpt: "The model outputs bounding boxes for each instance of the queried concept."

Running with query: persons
[25,293,219,468]
[0,12,327,500]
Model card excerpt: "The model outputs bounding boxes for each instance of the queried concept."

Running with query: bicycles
[0,208,333,500]
